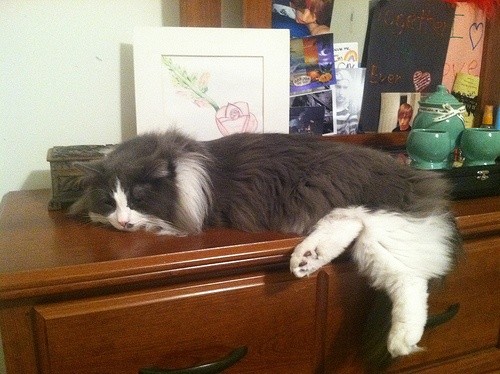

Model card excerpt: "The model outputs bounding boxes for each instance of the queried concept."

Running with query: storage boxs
[47,144,121,212]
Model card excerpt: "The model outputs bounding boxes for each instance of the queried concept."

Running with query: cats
[66,127,461,370]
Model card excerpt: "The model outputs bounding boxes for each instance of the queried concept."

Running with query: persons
[391,104,414,131]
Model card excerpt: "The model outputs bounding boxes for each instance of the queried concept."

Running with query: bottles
[480,106,493,128]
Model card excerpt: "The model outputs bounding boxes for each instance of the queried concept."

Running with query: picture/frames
[133,27,289,140]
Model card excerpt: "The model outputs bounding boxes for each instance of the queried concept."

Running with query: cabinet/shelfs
[0,130,500,374]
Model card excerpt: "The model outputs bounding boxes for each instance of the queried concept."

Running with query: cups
[460,128,500,166]
[406,129,451,170]
